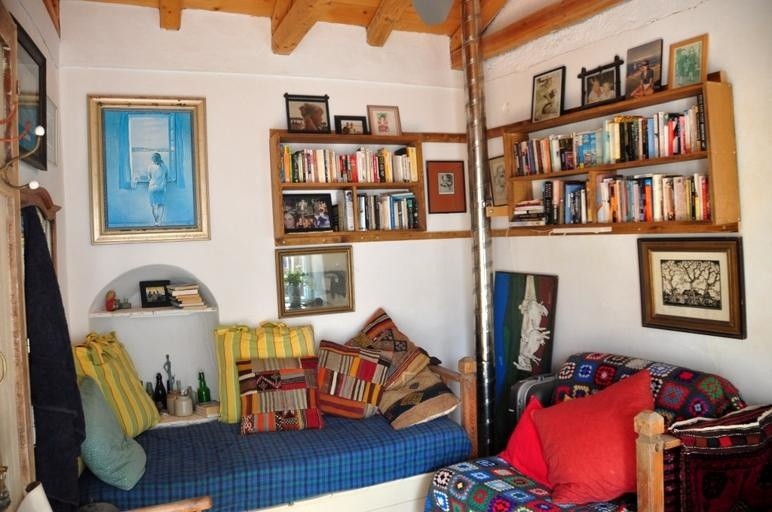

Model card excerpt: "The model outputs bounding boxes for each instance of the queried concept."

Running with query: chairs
[415,349,771,511]
[19,477,215,512]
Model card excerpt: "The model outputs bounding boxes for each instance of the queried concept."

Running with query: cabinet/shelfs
[482,69,746,240]
[91,304,222,428]
[266,123,472,249]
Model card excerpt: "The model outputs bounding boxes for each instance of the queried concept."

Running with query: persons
[677,46,696,85]
[630,58,654,99]
[343,122,356,135]
[588,76,614,104]
[147,153,168,227]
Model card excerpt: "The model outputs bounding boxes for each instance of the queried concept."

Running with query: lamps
[1,124,46,191]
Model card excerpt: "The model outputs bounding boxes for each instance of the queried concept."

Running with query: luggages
[505,370,560,436]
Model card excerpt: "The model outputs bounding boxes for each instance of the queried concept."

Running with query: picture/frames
[663,32,712,93]
[636,236,749,341]
[85,90,214,248]
[487,269,561,425]
[12,14,47,172]
[486,152,509,209]
[278,191,337,234]
[333,114,368,136]
[274,243,357,321]
[364,102,403,137]
[576,53,625,110]
[138,275,171,308]
[283,92,333,136]
[424,158,468,217]
[527,64,568,124]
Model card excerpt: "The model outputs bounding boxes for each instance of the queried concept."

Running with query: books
[279,144,418,231]
[166,283,208,310]
[508,92,711,229]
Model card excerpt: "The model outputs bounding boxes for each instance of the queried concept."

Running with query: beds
[19,177,483,512]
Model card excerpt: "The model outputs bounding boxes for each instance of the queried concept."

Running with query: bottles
[168,381,197,417]
[197,370,211,401]
[145,381,153,396]
[105,297,132,311]
[154,373,168,409]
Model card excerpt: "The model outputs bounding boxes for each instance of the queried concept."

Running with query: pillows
[373,363,463,432]
[233,352,326,438]
[74,326,168,439]
[523,365,660,507]
[308,335,394,420]
[492,391,553,492]
[73,372,154,493]
[208,317,324,424]
[341,302,442,392]
